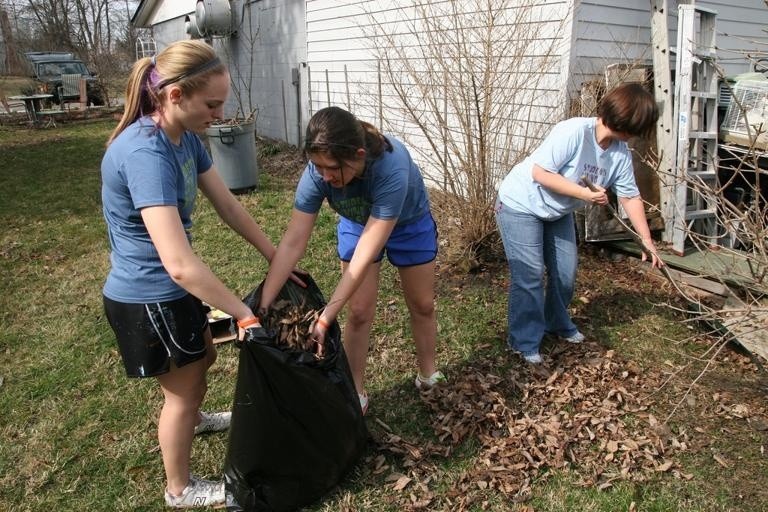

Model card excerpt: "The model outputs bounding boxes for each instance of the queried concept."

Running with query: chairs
[0,74,82,129]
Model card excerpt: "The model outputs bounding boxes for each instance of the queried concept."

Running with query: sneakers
[558,329,585,343]
[194,411,233,436]
[415,370,449,390]
[165,476,225,508]
[519,354,541,363]
[358,391,368,416]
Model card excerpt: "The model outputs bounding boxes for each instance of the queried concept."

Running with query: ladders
[651,0,720,255]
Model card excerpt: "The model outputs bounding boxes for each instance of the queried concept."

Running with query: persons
[494,83,665,363]
[100,40,308,508]
[258,107,447,416]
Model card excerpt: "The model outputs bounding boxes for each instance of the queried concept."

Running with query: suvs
[22,49,106,109]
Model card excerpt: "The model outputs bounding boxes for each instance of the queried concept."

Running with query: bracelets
[316,318,330,330]
[237,317,260,329]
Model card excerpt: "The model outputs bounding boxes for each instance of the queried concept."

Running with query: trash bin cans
[206,119,257,191]
